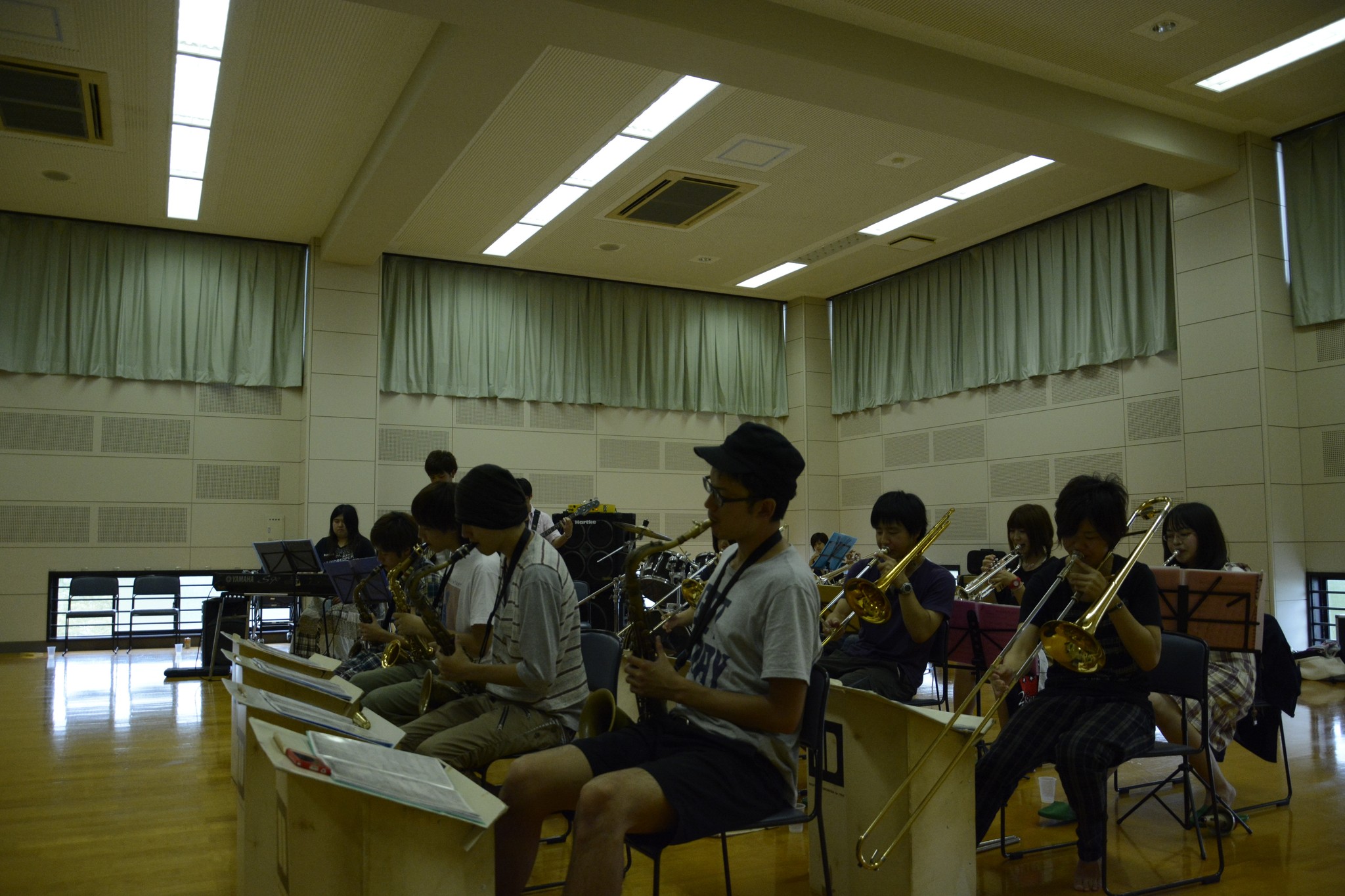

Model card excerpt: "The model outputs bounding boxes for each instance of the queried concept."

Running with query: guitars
[539,496,600,537]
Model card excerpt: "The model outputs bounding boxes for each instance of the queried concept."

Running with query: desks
[207,592,337,681]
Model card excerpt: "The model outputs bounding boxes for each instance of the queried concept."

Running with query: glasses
[702,475,748,505]
[1162,532,1194,540]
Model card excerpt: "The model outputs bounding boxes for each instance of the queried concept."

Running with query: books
[306,730,486,826]
[252,657,352,700]
[248,639,332,670]
[260,689,393,748]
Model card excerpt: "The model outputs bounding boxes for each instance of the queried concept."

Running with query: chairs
[999,626,1226,896]
[517,626,633,895]
[910,617,951,715]
[1110,691,1293,813]
[621,664,835,896]
[126,574,182,654]
[62,576,120,658]
[573,580,593,629]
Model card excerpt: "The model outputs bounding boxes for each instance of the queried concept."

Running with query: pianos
[208,572,336,681]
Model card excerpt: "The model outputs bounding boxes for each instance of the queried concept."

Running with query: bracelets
[564,532,572,538]
[1106,600,1123,614]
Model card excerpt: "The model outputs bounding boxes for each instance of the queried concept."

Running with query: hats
[692,421,806,501]
[454,464,528,530]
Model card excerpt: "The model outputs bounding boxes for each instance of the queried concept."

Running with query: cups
[1038,776,1056,803]
[175,644,183,655]
[47,646,56,657]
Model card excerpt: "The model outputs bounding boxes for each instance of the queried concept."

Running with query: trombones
[820,507,955,648]
[854,495,1173,871]
[616,551,725,640]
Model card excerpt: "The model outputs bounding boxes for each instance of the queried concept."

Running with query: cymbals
[614,521,673,541]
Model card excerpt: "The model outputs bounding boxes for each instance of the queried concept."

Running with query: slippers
[1038,801,1078,821]
[1189,805,1250,824]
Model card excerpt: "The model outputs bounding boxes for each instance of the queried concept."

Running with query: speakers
[203,597,250,667]
[552,512,636,631]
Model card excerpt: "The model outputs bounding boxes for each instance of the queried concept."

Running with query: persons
[808,532,843,585]
[393,463,591,772]
[821,491,956,701]
[494,422,822,895]
[424,450,458,482]
[661,531,738,633]
[976,473,1163,892]
[334,510,449,682]
[516,478,573,549]
[349,479,502,728]
[289,504,388,660]
[980,504,1060,719]
[1037,503,1258,827]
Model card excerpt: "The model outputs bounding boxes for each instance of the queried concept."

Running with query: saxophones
[347,564,385,661]
[409,541,476,715]
[578,519,712,741]
[381,542,434,669]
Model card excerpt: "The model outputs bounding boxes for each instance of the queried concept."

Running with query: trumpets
[812,548,880,585]
[955,544,1024,602]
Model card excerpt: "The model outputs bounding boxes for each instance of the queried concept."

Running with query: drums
[694,551,719,581]
[638,548,694,607]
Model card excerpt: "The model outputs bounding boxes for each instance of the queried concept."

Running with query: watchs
[897,582,912,594]
[1009,577,1020,590]
